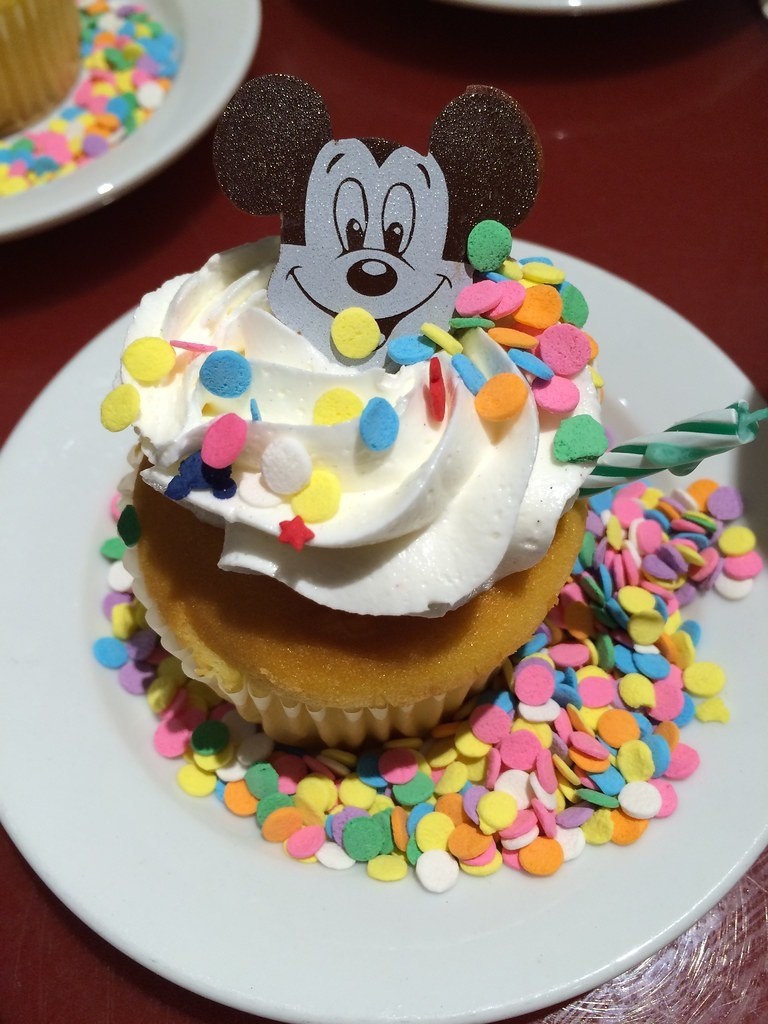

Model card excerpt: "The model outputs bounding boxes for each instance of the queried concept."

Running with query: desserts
[100,72,768,757]
[0,0,86,138]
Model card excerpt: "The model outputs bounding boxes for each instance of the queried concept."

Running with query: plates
[0,0,266,240]
[0,232,768,1024]
